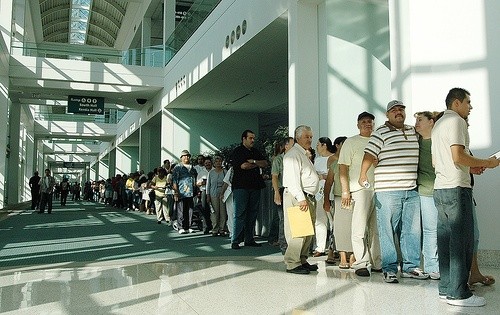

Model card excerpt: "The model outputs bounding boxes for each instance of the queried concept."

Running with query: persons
[29,100,495,292]
[430,87,500,308]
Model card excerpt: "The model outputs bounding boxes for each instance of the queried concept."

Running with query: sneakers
[400,268,429,279]
[382,271,399,283]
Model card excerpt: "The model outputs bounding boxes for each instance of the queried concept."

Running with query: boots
[146,208,153,215]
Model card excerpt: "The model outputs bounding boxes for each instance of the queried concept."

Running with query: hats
[180,150,192,158]
[357,110,376,121]
[386,99,407,113]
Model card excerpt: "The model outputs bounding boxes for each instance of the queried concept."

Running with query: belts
[303,190,316,200]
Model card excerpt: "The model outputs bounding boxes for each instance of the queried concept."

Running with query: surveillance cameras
[135,99,147,104]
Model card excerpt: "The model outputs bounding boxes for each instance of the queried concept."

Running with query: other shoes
[61,202,66,206]
[212,229,225,237]
[354,267,382,277]
[311,251,325,257]
[178,228,196,234]
[286,264,310,274]
[37,210,52,214]
[232,243,240,249]
[439,292,487,307]
[302,261,318,271]
[173,220,178,230]
[468,275,495,291]
[157,220,170,224]
[244,241,262,248]
[424,271,440,280]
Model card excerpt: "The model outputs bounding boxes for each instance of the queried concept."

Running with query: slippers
[339,262,350,269]
[325,257,336,264]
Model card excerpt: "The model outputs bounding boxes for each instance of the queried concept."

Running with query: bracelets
[254,159,257,163]
[343,192,350,194]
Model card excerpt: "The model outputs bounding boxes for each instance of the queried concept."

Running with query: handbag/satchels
[149,189,156,202]
